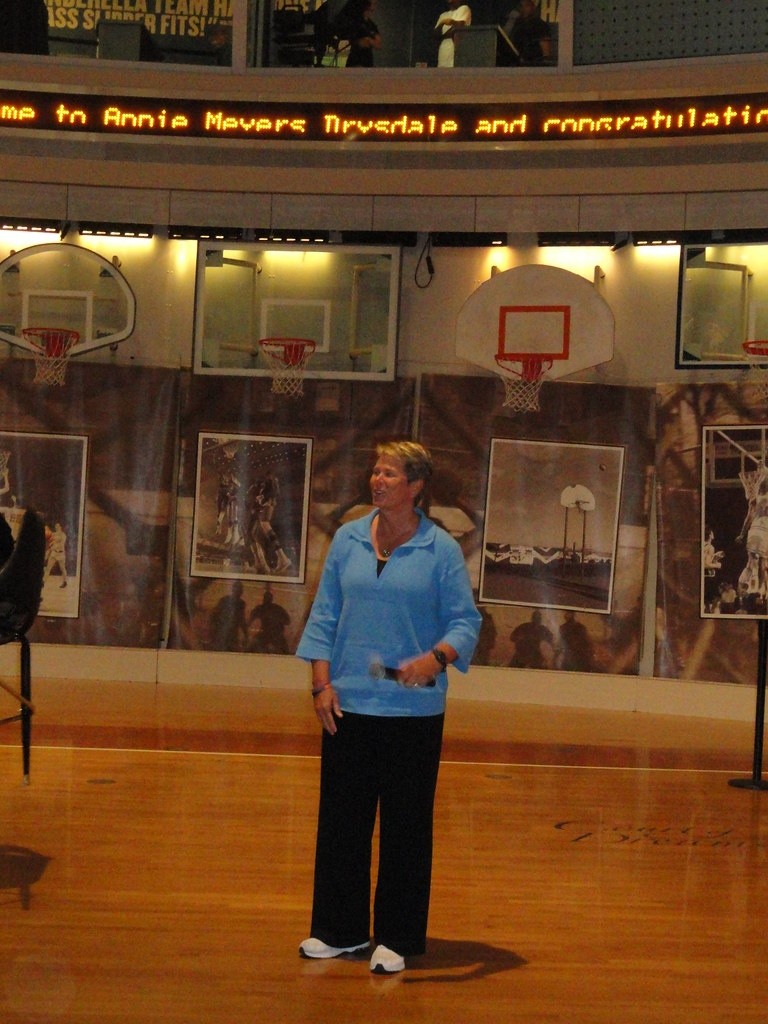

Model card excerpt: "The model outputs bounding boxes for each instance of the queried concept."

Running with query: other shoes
[59,581,67,588]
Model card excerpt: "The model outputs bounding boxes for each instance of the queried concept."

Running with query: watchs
[432,649,447,672]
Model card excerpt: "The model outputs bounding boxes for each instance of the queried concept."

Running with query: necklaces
[379,516,415,557]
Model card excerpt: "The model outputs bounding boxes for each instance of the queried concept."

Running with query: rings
[413,682,418,687]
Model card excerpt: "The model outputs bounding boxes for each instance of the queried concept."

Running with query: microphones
[370,662,437,689]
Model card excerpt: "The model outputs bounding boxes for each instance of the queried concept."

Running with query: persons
[0,466,68,588]
[296,442,481,974]
[434,0,471,68]
[498,0,552,66]
[705,479,768,616]
[0,0,49,54]
[344,5,381,66]
[205,580,592,675]
[196,454,298,576]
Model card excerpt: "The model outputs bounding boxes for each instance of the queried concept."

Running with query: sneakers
[370,944,406,974]
[298,938,370,958]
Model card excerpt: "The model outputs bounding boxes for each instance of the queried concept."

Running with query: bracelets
[312,684,332,697]
